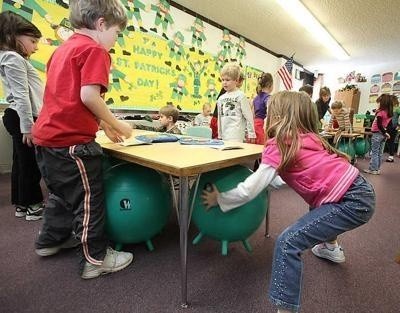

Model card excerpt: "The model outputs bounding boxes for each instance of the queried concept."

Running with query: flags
[276,55,293,91]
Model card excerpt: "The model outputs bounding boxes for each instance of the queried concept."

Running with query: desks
[319,127,382,156]
[91,126,274,307]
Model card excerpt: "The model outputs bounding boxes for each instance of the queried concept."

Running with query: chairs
[184,124,211,140]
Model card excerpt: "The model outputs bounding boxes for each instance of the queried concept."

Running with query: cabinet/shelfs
[334,88,361,120]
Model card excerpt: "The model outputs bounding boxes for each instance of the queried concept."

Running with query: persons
[328,100,357,166]
[29,0,136,282]
[314,86,333,131]
[0,9,54,222]
[193,102,212,128]
[198,89,378,312]
[300,84,312,96]
[210,71,244,140]
[361,93,400,175]
[216,62,257,144]
[158,105,182,135]
[250,70,274,144]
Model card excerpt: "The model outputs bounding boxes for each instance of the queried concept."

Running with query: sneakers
[363,168,381,175]
[15,205,27,217]
[81,246,133,279]
[311,244,346,264]
[385,156,395,163]
[34,232,80,256]
[25,203,47,221]
